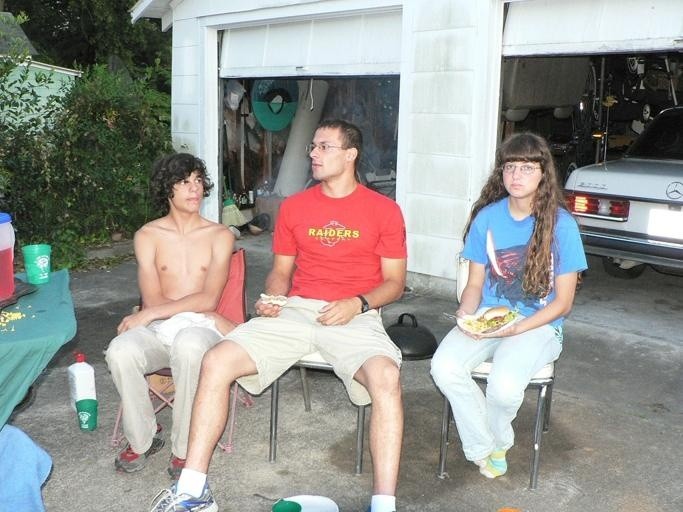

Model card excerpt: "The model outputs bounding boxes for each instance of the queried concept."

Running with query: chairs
[263,302,386,475]
[106,248,255,454]
[434,242,565,493]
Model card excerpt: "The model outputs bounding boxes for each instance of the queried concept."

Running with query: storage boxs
[142,371,175,397]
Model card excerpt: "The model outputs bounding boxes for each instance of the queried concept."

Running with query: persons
[154,121,407,511]
[429,132,586,478]
[106,154,234,482]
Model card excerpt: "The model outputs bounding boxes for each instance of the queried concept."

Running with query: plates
[455,313,525,335]
[283,495,341,512]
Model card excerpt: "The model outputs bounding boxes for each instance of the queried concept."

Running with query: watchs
[358,294,369,313]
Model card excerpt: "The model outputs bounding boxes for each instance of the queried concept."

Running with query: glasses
[501,162,542,174]
[305,143,345,154]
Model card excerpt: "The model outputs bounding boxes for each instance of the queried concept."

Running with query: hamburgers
[482,306,516,331]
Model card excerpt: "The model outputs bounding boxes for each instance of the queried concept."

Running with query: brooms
[221,176,249,228]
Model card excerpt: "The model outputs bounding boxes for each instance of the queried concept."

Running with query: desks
[0,268,79,418]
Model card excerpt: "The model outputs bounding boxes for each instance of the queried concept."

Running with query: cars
[564,105,683,280]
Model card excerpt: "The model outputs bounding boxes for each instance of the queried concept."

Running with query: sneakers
[150,479,220,512]
[114,423,167,472]
[167,452,186,484]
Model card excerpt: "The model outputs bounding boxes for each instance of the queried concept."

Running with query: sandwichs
[260,292,291,308]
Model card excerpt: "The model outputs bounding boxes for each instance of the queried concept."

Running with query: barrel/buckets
[272,495,339,512]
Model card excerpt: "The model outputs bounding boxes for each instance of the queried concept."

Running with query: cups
[270,497,303,512]
[75,398,98,432]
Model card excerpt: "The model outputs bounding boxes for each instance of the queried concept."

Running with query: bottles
[0,212,16,303]
[64,353,98,413]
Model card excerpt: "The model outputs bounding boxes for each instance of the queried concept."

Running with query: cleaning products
[68,353,97,413]
